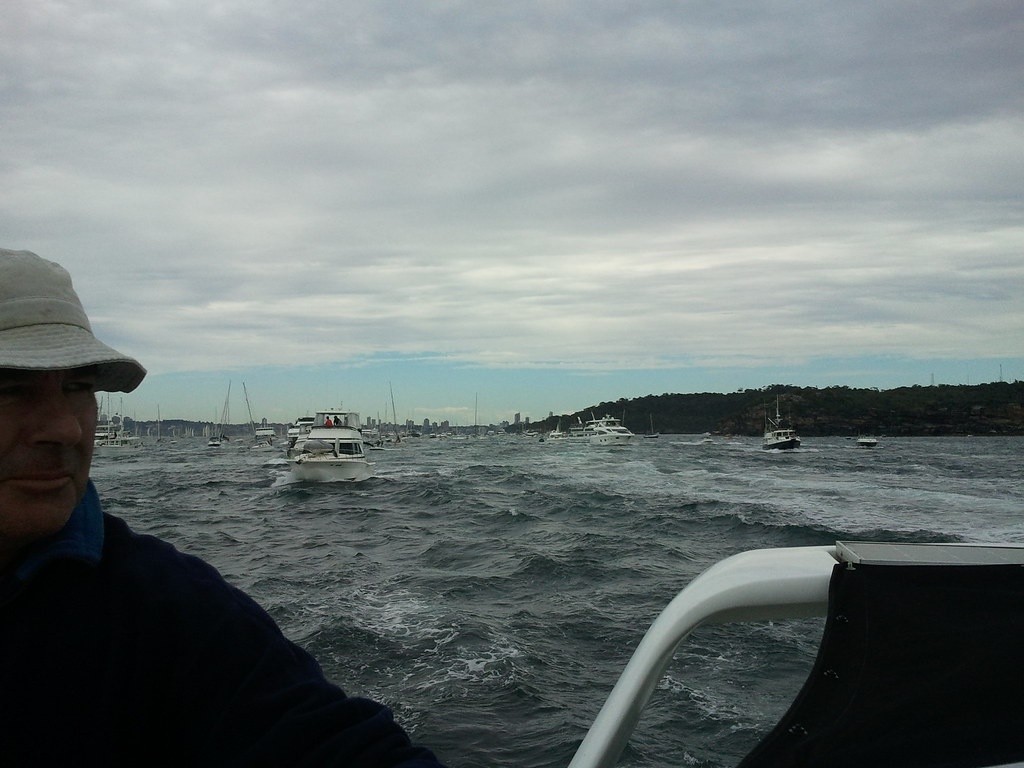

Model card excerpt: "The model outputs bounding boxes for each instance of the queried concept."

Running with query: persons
[333,416,342,425]
[0,249,445,768]
[325,416,332,425]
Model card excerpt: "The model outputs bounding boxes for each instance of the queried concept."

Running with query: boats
[856,435,878,448]
[700,437,712,445]
[762,394,801,450]
[286,407,376,482]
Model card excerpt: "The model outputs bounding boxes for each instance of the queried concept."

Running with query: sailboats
[92,378,637,457]
[644,414,659,439]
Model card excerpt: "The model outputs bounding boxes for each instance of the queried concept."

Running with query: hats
[0,249,148,395]
